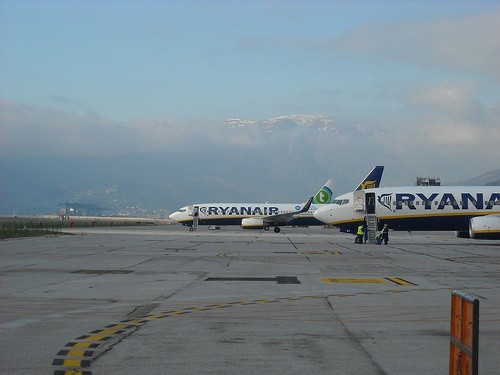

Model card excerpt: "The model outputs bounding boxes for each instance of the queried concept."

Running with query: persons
[363,225,371,244]
[357,224,365,244]
[382,224,389,244]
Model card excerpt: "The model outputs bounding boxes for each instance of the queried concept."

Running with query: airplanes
[168,176,336,233]
[12,207,75,221]
[313,165,500,244]
